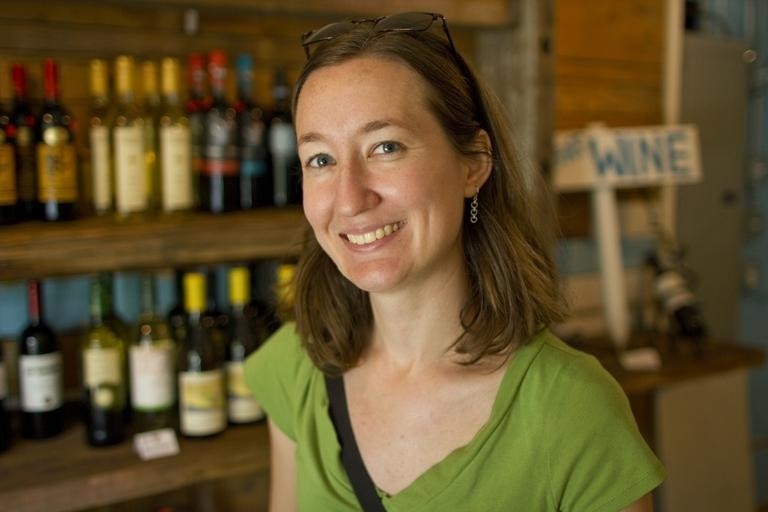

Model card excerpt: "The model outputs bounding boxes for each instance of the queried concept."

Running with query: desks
[570,334,768,512]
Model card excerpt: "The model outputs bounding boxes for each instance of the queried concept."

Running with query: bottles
[645,248,708,356]
[268,262,300,330]
[129,271,179,459]
[220,267,269,427]
[73,266,127,448]
[169,273,224,439]
[0,48,303,224]
[15,278,65,443]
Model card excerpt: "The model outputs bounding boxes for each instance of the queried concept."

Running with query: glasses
[301,11,466,74]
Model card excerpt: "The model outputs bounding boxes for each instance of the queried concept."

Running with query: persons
[240,18,676,512]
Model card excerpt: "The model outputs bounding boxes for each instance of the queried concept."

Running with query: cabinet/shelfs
[0,0,554,512]
